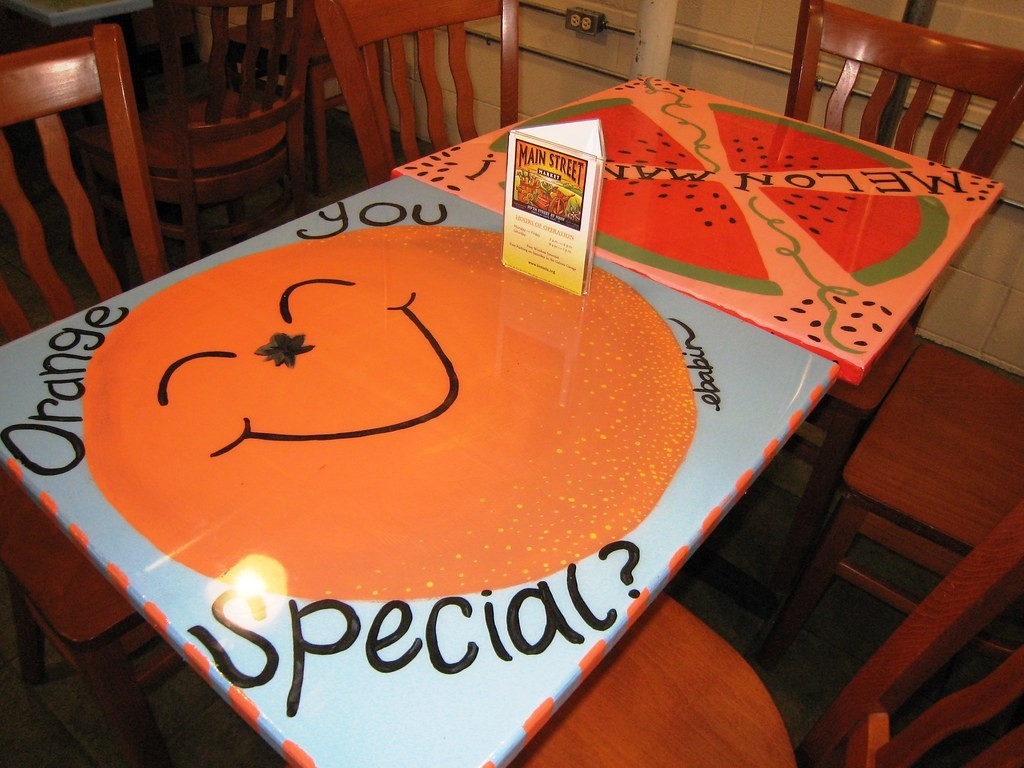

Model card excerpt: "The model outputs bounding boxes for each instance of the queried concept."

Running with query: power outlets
[565,6,607,37]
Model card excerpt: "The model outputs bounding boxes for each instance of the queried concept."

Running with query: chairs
[515,503,1024,768]
[75,0,314,290]
[228,17,396,196]
[777,1,1024,571]
[0,21,171,344]
[755,342,1024,668]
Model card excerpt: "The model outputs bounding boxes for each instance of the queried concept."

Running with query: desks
[387,71,1004,385]
[0,170,838,768]
